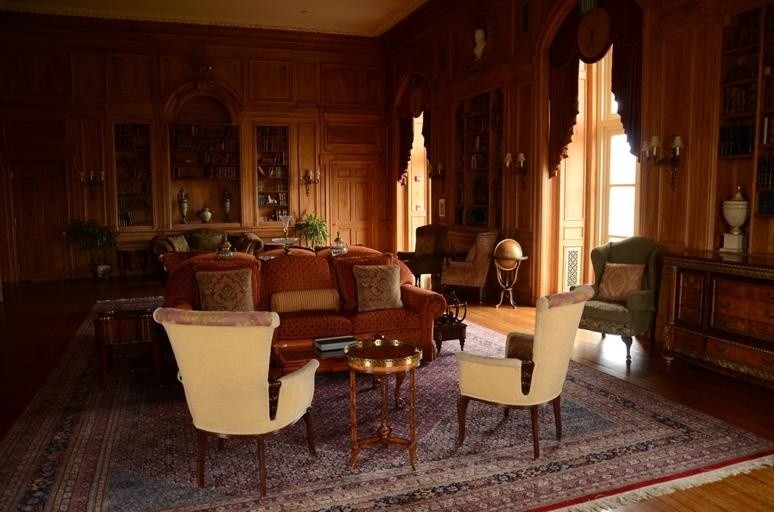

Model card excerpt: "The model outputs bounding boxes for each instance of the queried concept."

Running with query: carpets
[1,296,774,511]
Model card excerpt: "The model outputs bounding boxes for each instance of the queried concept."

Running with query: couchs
[161,246,449,367]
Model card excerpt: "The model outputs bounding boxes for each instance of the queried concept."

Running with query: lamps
[299,168,320,196]
[640,134,680,190]
[438,163,444,178]
[78,167,108,198]
[504,154,528,182]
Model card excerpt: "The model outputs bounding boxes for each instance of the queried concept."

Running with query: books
[255,124,289,222]
[313,334,358,353]
[113,125,154,228]
[172,126,239,181]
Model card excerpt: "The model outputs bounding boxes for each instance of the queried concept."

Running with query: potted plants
[65,217,118,279]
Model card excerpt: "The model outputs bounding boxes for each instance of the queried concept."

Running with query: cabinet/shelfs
[105,111,297,232]
[656,252,773,394]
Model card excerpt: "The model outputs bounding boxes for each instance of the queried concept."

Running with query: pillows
[175,255,403,313]
[593,261,644,302]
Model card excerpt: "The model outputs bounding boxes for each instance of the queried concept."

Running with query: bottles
[515,153,526,168]
[503,154,512,168]
[118,189,125,209]
[332,231,348,257]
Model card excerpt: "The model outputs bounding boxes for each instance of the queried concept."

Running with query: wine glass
[279,216,292,241]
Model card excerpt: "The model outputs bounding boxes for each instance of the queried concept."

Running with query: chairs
[396,224,444,287]
[455,287,591,457]
[573,235,667,377]
[151,307,318,497]
[152,230,263,254]
[442,227,494,303]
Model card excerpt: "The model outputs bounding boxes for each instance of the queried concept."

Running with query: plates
[272,237,297,244]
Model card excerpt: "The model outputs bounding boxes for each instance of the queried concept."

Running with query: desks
[345,341,421,471]
[99,308,161,385]
